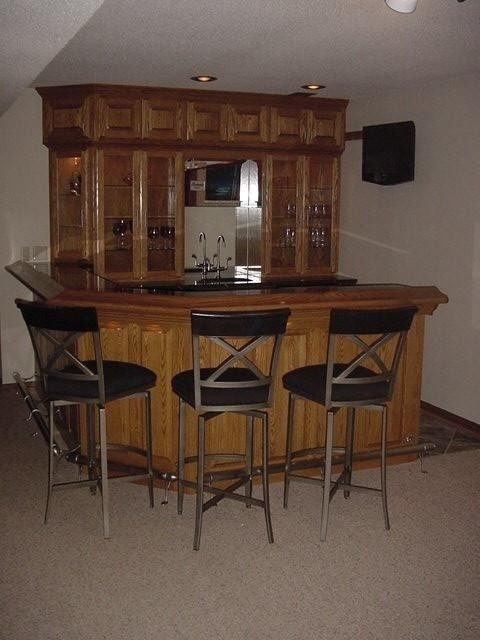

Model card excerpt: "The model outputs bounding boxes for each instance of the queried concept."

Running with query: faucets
[202,258,211,272]
[216,235,226,273]
[198,232,207,274]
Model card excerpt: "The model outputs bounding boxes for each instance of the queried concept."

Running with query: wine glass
[111,222,175,251]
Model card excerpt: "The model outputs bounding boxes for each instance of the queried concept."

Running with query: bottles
[282,203,330,248]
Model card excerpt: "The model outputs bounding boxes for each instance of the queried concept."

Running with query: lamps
[385,0,417,13]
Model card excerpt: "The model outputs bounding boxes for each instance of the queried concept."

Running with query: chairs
[171,303,292,551]
[283,308,419,541]
[15,298,158,540]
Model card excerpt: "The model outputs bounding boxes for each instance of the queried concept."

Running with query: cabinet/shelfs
[258,157,338,277]
[35,87,348,152]
[48,145,184,279]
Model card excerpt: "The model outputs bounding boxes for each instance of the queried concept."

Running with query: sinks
[243,267,262,272]
[184,267,225,273]
[196,277,253,284]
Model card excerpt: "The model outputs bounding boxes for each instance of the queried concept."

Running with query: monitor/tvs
[361,120,415,186]
